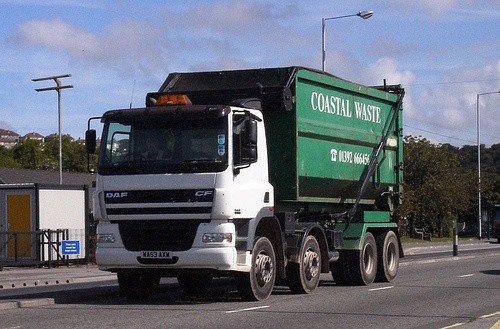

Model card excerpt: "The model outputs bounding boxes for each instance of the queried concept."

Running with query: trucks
[84,66,406,301]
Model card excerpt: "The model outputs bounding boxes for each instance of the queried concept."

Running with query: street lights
[321,8,373,72]
[476,91,500,240]
